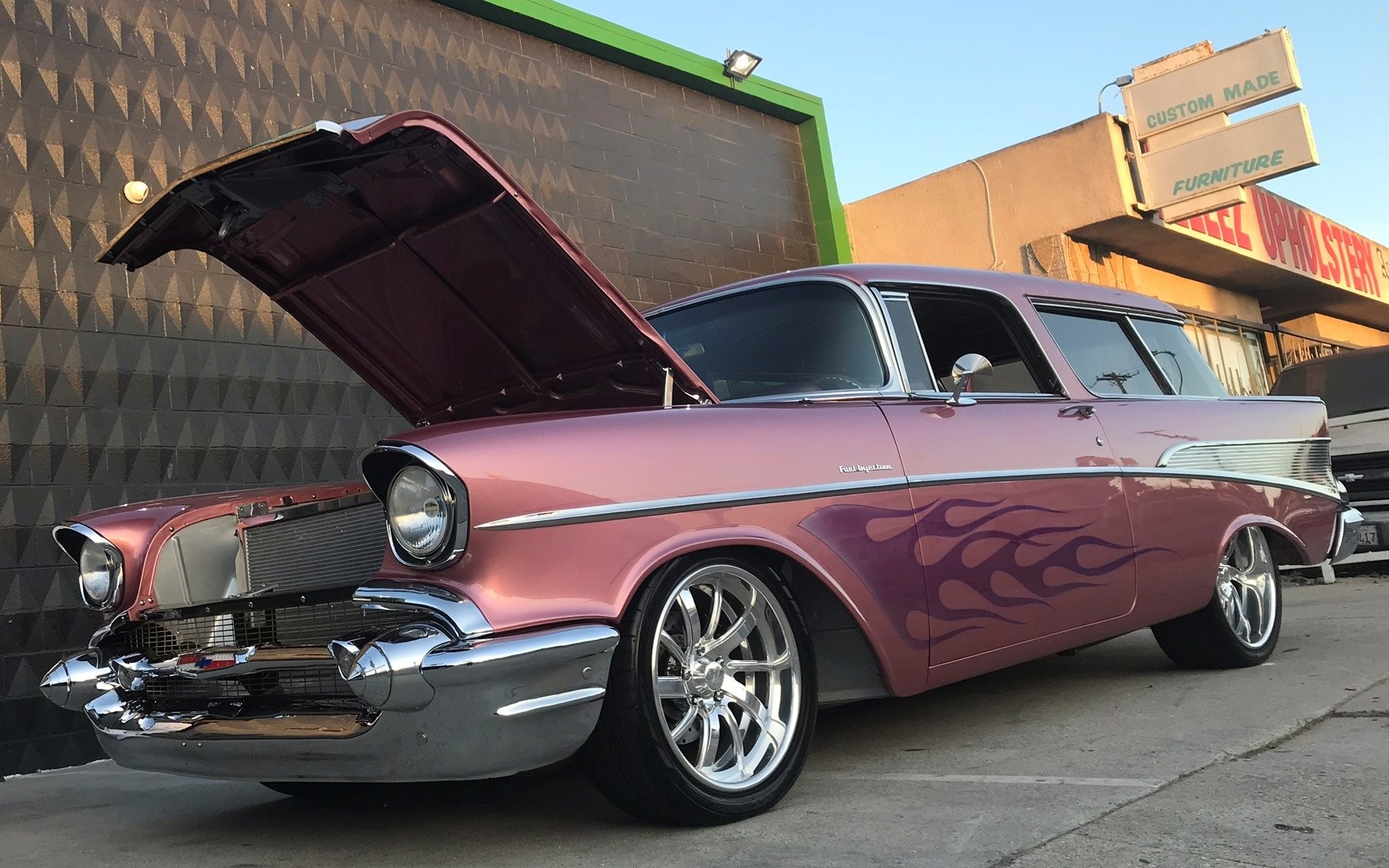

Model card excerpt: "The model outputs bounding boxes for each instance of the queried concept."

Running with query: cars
[1267,343,1389,578]
[36,107,1366,832]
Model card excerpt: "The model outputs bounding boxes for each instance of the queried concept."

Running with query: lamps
[722,49,762,79]
[1099,75,1134,113]
